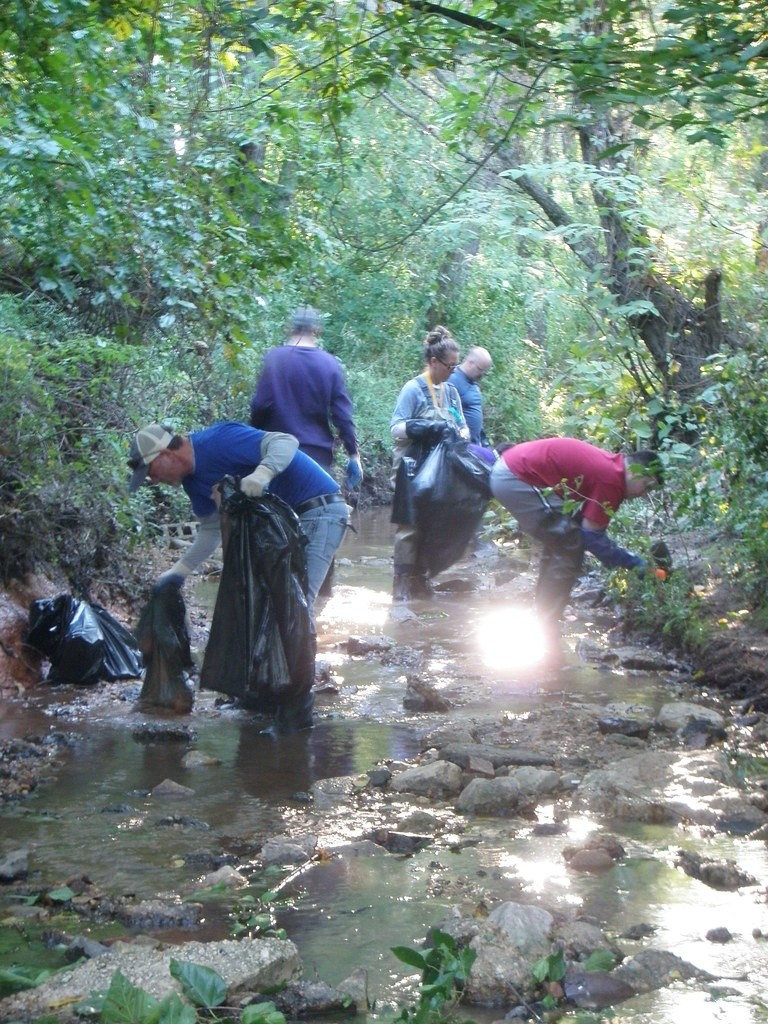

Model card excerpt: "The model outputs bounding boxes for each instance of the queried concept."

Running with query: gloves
[406,418,458,438]
[149,561,190,599]
[240,465,273,499]
[345,455,363,490]
[581,527,650,579]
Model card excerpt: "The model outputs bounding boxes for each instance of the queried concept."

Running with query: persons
[489,437,666,638]
[125,420,352,733]
[445,347,493,550]
[249,306,364,599]
[390,326,470,603]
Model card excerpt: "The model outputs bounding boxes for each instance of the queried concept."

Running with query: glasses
[435,355,460,371]
[474,363,486,374]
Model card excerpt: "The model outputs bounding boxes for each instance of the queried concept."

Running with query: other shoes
[219,696,248,711]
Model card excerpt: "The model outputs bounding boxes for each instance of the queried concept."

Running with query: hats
[128,424,177,492]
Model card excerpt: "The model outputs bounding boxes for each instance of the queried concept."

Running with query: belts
[294,494,345,514]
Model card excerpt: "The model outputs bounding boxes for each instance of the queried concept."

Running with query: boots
[392,561,415,601]
